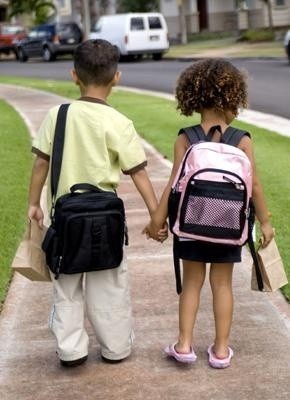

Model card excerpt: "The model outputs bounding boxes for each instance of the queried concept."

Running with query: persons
[25,38,168,370]
[141,55,274,370]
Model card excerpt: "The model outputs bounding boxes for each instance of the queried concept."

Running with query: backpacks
[169,142,255,247]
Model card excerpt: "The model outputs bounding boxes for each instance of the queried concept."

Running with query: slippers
[165,340,234,369]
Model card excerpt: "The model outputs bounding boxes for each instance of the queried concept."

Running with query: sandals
[60,355,123,367]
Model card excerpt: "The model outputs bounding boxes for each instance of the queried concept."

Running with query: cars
[0,22,26,56]
[15,21,83,63]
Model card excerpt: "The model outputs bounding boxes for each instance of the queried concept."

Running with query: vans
[87,11,170,60]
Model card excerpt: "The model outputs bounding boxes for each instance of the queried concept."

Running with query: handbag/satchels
[42,184,123,275]
[11,219,53,282]
[251,228,288,292]
